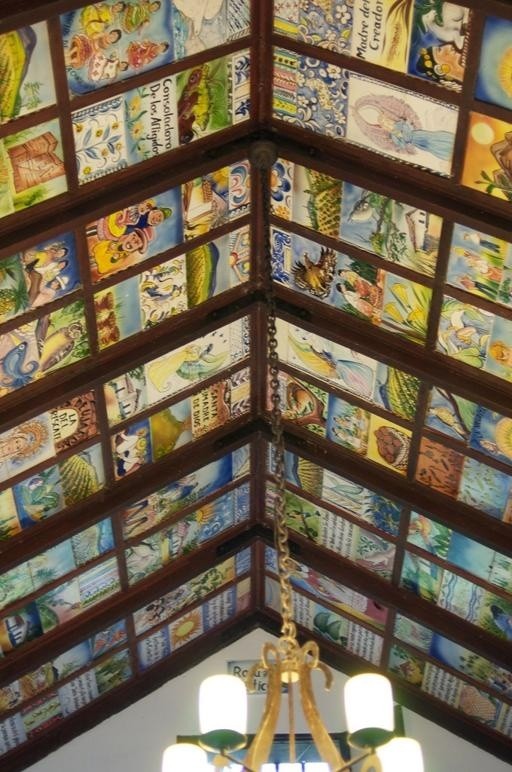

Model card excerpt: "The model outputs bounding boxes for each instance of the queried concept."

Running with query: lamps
[160,140,425,772]
